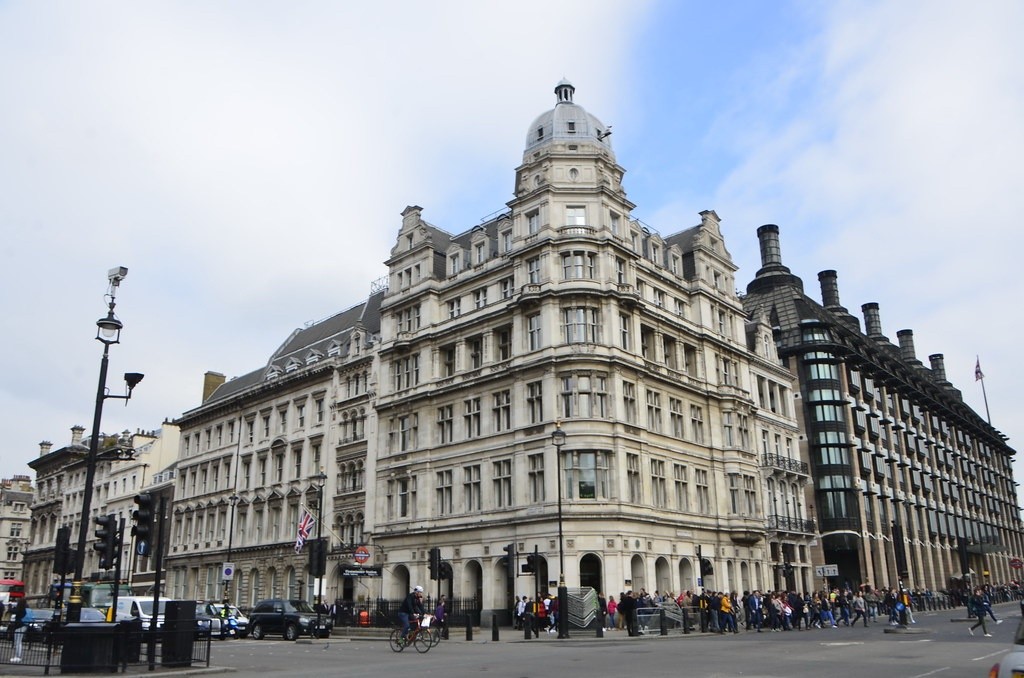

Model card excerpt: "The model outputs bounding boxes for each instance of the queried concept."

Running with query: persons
[968,588,993,637]
[1015,598,1024,644]
[434,595,449,640]
[512,595,560,638]
[5,598,27,662]
[220,603,232,632]
[598,588,872,635]
[398,586,431,644]
[865,580,1024,625]
[319,598,340,627]
[0,600,34,624]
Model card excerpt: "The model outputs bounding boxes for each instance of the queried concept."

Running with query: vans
[116,596,171,629]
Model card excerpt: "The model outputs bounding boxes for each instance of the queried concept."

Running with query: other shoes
[907,620,916,624]
[996,620,1003,625]
[889,621,899,626]
[10,657,22,662]
[757,629,764,632]
[831,624,838,628]
[409,630,420,636]
[769,625,784,632]
[400,635,406,645]
[544,625,550,634]
[968,628,974,636]
[602,627,607,631]
[809,622,826,628]
[607,626,618,632]
[550,628,557,633]
[799,627,811,630]
[984,634,992,638]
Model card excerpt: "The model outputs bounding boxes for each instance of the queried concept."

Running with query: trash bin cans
[120,616,142,664]
[60,622,120,673]
[162,601,196,667]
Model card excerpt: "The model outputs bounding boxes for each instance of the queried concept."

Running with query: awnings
[957,543,1011,556]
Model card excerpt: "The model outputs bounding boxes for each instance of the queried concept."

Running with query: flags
[294,509,316,555]
[976,359,984,381]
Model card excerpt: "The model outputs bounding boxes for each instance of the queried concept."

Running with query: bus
[49,579,132,622]
[0,579,25,628]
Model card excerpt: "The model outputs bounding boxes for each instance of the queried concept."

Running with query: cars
[990,616,1024,678]
[60,608,106,622]
[195,600,250,639]
[6,608,54,642]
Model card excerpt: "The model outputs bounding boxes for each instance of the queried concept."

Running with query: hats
[416,585,424,592]
[902,589,906,592]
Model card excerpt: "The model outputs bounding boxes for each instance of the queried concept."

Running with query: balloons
[1009,558,1023,569]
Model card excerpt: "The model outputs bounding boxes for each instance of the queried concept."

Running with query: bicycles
[390,613,440,653]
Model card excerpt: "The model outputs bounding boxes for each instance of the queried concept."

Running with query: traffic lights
[701,558,711,575]
[131,494,154,538]
[503,543,514,567]
[94,514,115,569]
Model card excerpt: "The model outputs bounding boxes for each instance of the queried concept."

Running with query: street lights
[308,465,327,639]
[221,492,240,605]
[65,296,145,624]
[552,418,571,638]
[22,540,31,581]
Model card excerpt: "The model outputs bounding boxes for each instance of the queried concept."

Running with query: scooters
[221,614,240,639]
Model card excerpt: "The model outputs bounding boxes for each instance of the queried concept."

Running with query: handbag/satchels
[803,603,810,613]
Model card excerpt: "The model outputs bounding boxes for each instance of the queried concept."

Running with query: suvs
[249,598,333,641]
[25,594,49,608]
